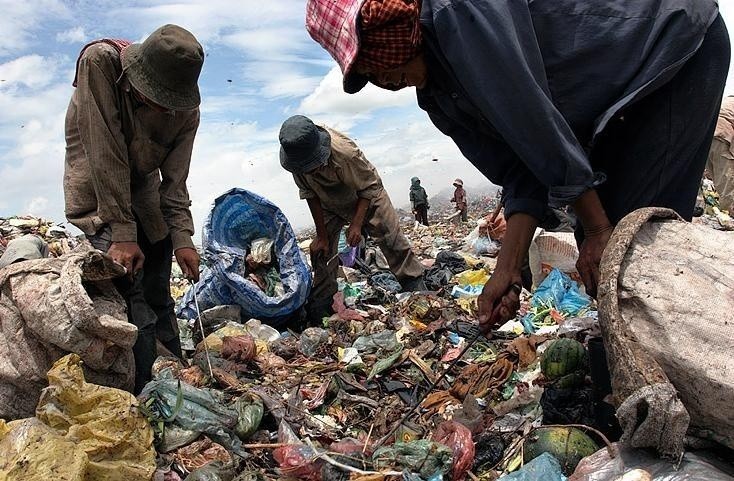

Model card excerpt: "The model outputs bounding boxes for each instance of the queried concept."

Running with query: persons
[410,177,430,226]
[706,93,733,217]
[451,178,467,222]
[308,0,731,440]
[279,116,425,322]
[65,20,204,395]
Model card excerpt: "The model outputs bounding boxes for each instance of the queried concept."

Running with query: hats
[307,0,368,93]
[279,115,332,171]
[453,178,464,187]
[120,25,204,109]
[411,178,420,184]
